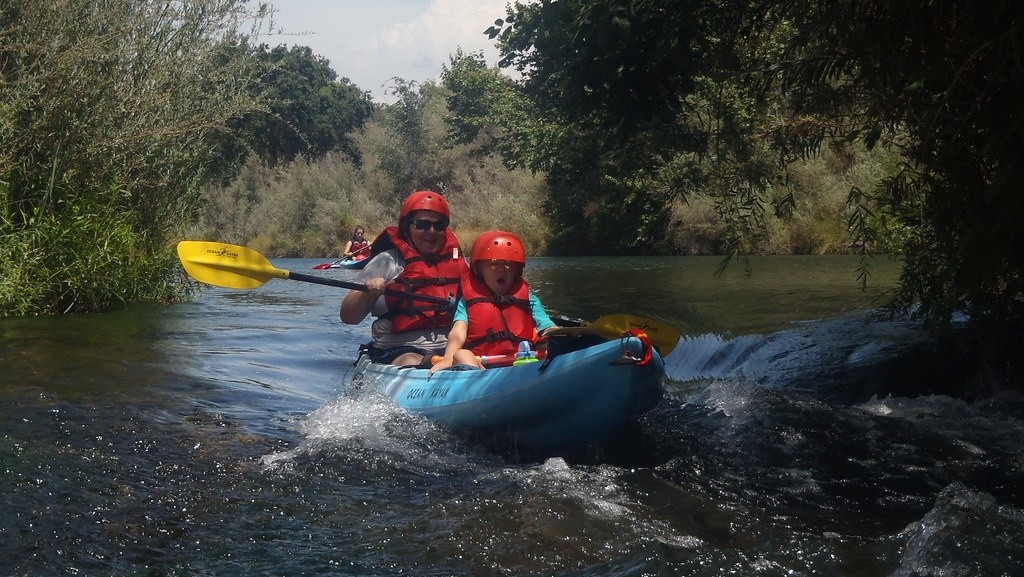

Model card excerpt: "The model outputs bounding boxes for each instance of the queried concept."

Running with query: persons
[427,230,560,380]
[343,224,371,261]
[339,191,470,369]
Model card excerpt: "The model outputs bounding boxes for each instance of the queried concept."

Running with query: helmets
[400,190,450,227]
[470,230,526,278]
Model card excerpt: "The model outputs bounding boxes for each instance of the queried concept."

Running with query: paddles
[313,242,373,270]
[177,240,681,359]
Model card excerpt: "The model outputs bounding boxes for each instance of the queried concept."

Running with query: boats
[339,258,371,269]
[347,328,666,463]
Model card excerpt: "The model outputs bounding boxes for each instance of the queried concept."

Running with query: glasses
[410,219,445,231]
[478,261,511,270]
